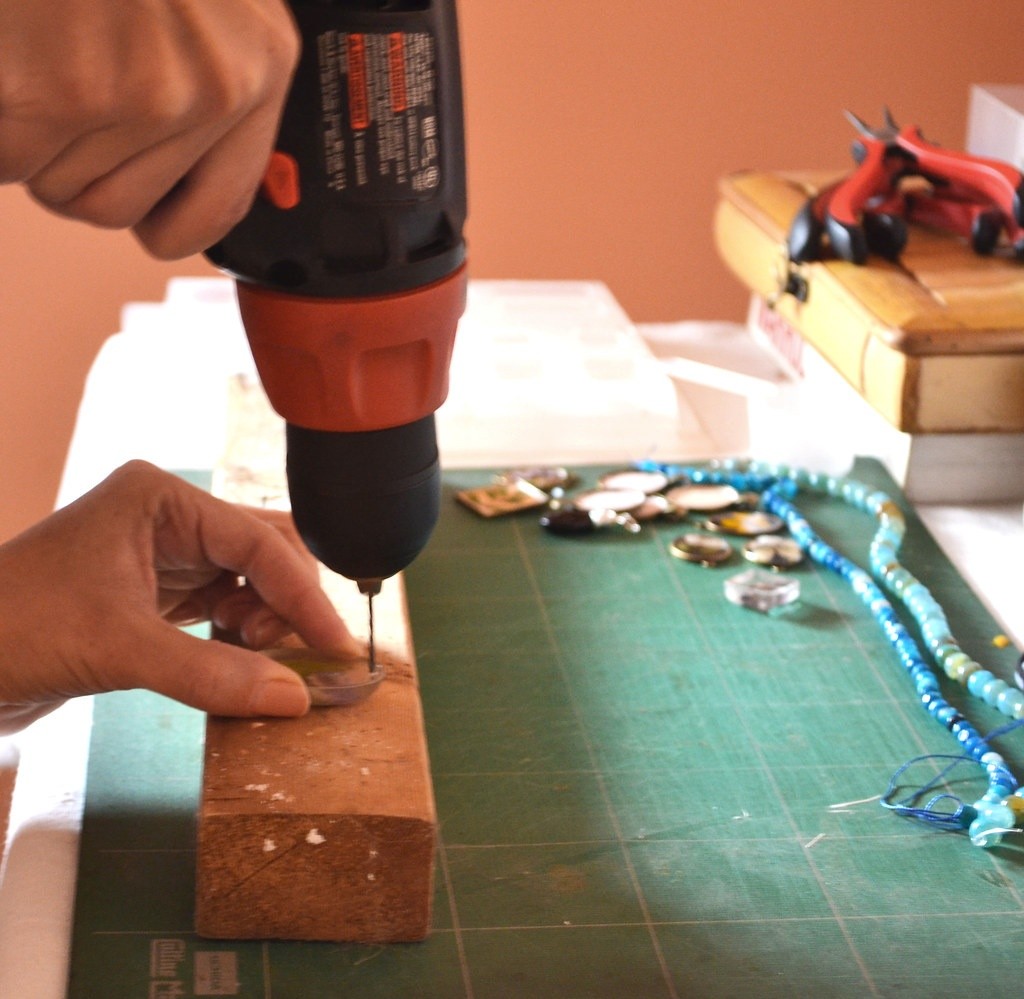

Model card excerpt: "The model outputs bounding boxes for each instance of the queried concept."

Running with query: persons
[0,0,372,743]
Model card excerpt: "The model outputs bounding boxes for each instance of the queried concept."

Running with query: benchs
[709,163,1023,438]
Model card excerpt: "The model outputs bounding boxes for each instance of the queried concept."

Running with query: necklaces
[636,459,1024,848]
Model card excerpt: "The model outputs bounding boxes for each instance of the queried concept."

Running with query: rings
[725,569,800,610]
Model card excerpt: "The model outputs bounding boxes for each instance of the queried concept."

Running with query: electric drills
[159,0,469,678]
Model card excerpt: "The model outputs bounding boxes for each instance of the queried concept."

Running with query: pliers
[786,102,1024,264]
